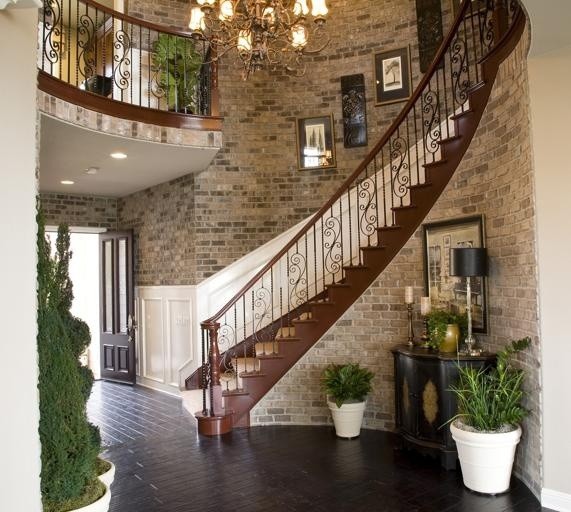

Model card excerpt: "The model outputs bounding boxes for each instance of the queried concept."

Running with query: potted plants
[319,361,377,440]
[151,34,204,115]
[421,309,468,353]
[436,331,535,496]
[49,220,116,487]
[36,191,111,512]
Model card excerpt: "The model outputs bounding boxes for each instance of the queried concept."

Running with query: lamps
[187,0,331,82]
[449,247,489,357]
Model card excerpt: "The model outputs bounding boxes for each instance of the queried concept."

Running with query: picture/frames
[295,111,337,171]
[373,43,413,107]
[450,0,487,25]
[421,213,489,336]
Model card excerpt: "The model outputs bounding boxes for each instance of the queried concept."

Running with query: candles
[404,286,414,304]
[420,296,431,316]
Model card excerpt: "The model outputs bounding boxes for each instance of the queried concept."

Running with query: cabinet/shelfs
[389,340,506,471]
